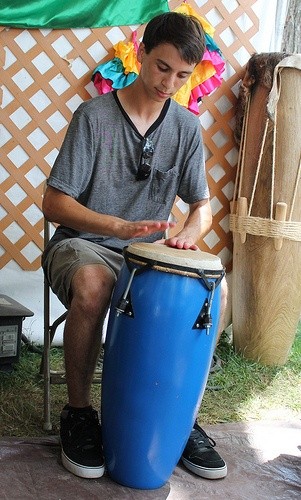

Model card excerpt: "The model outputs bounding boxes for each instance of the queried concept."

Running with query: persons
[41,12,228,480]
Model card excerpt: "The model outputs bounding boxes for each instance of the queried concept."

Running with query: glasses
[135,136,154,181]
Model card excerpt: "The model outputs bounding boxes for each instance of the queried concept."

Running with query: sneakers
[181,417,227,478]
[59,403,105,478]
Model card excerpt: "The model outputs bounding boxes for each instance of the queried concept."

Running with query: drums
[229,49,301,369]
[99,243,228,492]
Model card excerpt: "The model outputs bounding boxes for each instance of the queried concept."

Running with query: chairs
[38,178,104,431]
